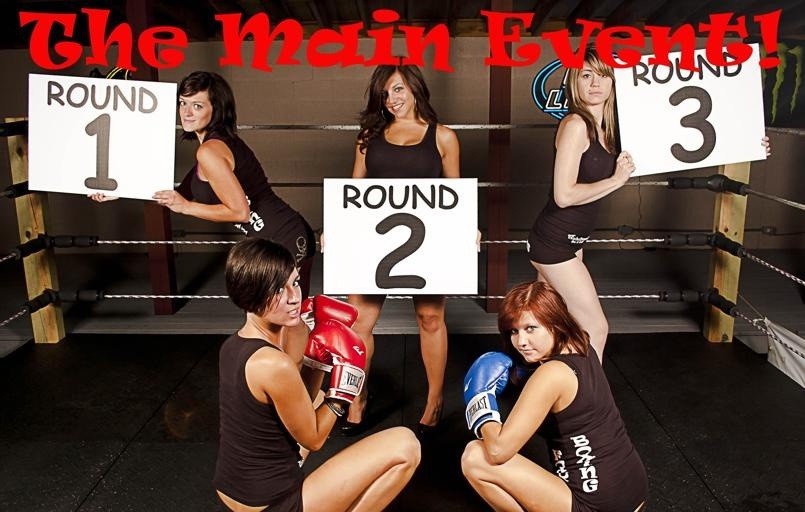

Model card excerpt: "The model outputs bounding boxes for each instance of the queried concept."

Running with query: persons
[86,42,772,512]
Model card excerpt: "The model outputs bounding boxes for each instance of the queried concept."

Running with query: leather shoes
[339,390,371,437]
[417,394,443,446]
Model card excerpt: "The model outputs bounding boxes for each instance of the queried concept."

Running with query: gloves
[463,351,539,440]
[300,294,358,372]
[309,318,366,404]
